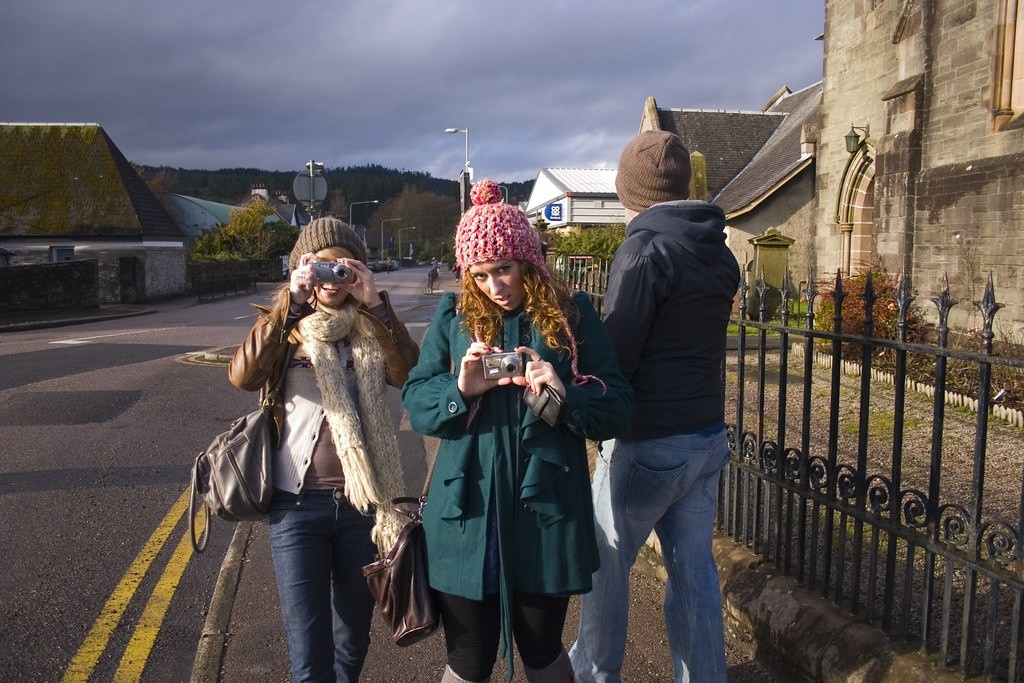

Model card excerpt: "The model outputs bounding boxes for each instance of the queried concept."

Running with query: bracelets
[288,306,302,319]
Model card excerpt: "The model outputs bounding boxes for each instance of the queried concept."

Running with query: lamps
[844,121,869,154]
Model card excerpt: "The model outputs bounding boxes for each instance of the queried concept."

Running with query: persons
[400,179,633,683]
[453,259,461,282]
[431,256,440,272]
[387,257,392,273]
[570,133,741,683]
[229,217,420,683]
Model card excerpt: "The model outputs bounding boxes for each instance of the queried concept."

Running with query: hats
[289,217,367,276]
[455,178,551,287]
[616,130,691,214]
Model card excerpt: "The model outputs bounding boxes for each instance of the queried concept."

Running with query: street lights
[380,218,403,260]
[443,128,469,175]
[349,200,379,227]
[398,226,415,267]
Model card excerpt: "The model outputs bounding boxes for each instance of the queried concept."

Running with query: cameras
[482,351,525,379]
[308,260,352,283]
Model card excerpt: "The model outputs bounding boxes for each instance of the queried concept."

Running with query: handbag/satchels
[362,496,444,647]
[187,344,291,553]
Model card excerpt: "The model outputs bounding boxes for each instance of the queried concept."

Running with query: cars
[367,260,400,273]
